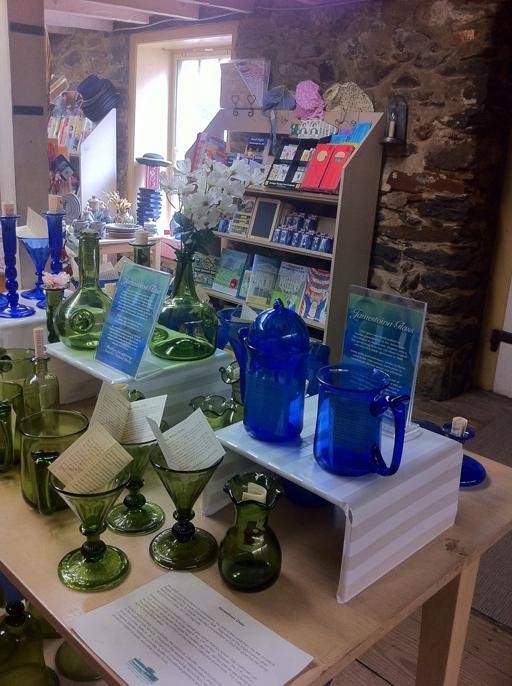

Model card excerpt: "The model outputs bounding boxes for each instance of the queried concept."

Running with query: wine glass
[149,447,224,573]
[48,460,133,592]
[105,418,169,537]
[17,224,65,301]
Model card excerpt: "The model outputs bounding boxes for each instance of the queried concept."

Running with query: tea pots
[217,308,331,443]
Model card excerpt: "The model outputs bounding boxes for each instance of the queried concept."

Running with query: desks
[0,347,512,686]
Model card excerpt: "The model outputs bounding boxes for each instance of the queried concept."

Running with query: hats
[136,153,171,167]
[76,75,120,123]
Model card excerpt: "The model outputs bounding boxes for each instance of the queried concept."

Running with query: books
[191,130,355,326]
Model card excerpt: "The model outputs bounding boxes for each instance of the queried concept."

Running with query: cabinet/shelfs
[183,107,386,368]
[49,108,119,220]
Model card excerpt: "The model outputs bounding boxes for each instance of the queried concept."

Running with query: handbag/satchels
[262,79,374,120]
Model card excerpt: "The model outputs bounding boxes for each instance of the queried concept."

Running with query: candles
[387,111,396,138]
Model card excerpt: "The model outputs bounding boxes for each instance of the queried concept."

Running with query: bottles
[53,231,113,350]
[189,361,244,432]
[218,474,282,593]
[148,250,218,362]
[22,327,60,435]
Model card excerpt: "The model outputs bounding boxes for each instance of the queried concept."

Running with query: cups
[17,409,89,516]
[313,364,410,478]
[0,345,35,474]
[73,220,104,240]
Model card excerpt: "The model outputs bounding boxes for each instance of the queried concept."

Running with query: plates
[62,193,81,224]
[106,222,144,238]
